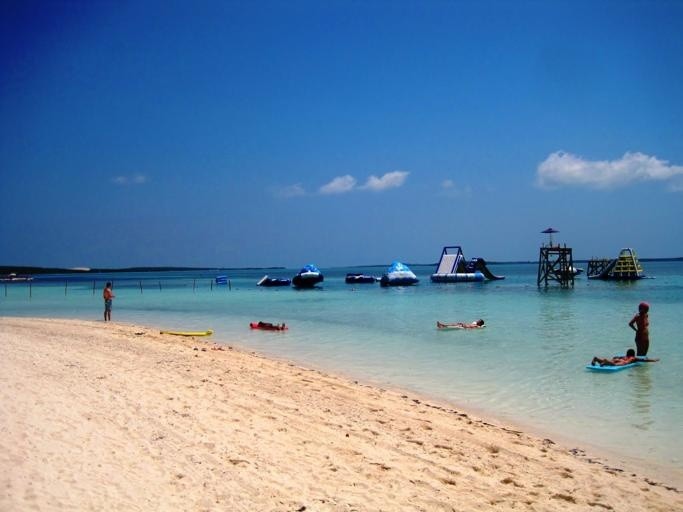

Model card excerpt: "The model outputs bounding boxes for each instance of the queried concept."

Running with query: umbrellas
[539,227,560,246]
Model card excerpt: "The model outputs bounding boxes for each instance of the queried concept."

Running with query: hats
[639,302,649,312]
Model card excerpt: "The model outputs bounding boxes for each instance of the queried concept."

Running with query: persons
[591,349,659,367]
[103,282,114,321]
[628,301,649,356]
[436,319,484,328]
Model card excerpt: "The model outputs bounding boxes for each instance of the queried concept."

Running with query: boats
[256,261,418,287]
